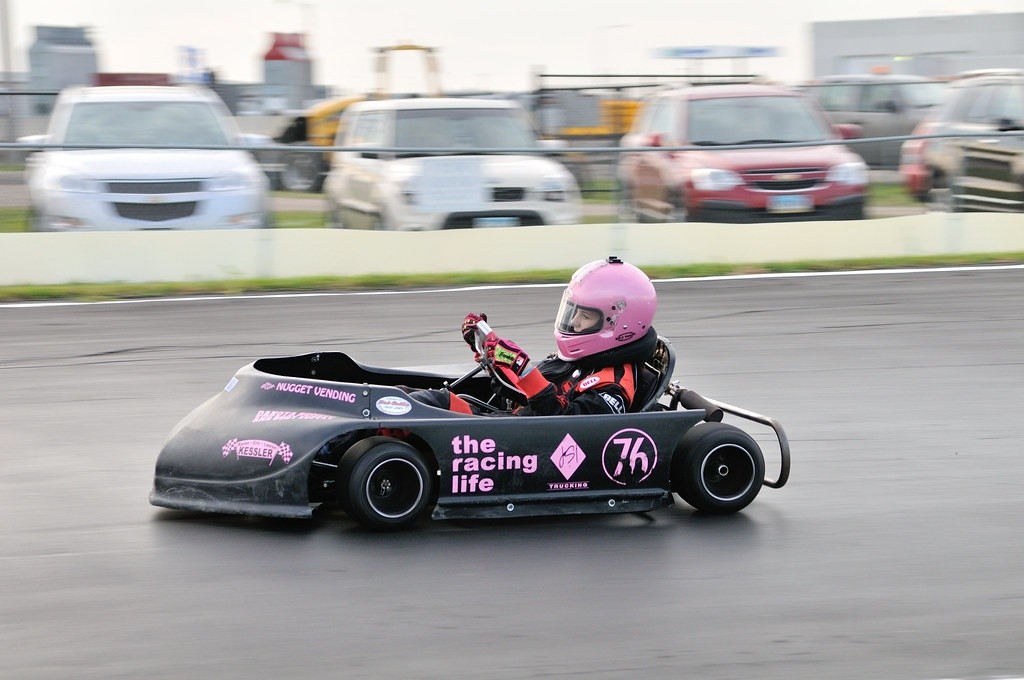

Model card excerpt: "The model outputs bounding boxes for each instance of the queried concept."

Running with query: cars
[803,76,949,167]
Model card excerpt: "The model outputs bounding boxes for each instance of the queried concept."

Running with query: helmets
[553,256,657,362]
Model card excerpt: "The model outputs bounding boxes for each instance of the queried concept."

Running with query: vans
[324,97,585,231]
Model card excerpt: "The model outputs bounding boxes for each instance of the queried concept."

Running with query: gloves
[461,312,488,362]
[486,337,530,376]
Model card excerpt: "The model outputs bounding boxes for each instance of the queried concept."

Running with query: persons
[408,256,658,418]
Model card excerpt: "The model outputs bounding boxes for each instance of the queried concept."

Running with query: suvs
[894,68,1024,212]
[16,83,275,234]
[612,84,875,221]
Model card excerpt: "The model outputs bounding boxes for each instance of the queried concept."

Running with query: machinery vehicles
[273,42,447,193]
